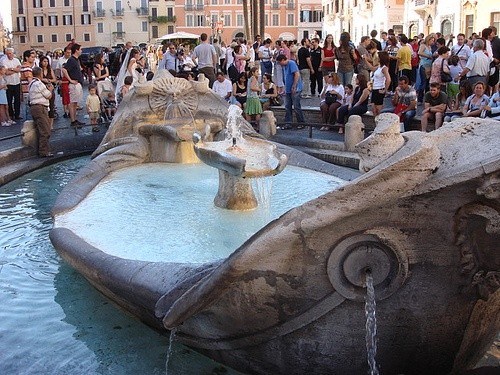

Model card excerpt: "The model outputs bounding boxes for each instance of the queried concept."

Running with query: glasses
[328,76,333,78]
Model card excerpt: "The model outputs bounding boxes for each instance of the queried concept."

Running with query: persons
[259,72,278,111]
[209,25,500,99]
[192,33,217,89]
[0,39,198,127]
[318,72,500,134]
[276,54,305,129]
[27,66,56,159]
[233,72,248,111]
[85,85,101,132]
[367,51,392,134]
[243,67,262,121]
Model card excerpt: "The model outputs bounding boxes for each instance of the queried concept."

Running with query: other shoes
[1,119,17,126]
[301,95,312,99]
[297,123,304,128]
[70,120,86,127]
[41,151,53,157]
[11,115,23,121]
[76,106,83,110]
[281,123,292,130]
[92,127,101,132]
[63,112,71,118]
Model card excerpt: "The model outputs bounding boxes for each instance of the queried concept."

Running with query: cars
[78,46,112,71]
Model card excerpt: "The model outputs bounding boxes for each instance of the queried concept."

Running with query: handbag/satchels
[325,91,337,105]
[394,102,409,122]
[440,58,453,82]
[272,93,284,106]
[448,55,459,66]
[264,61,271,68]
